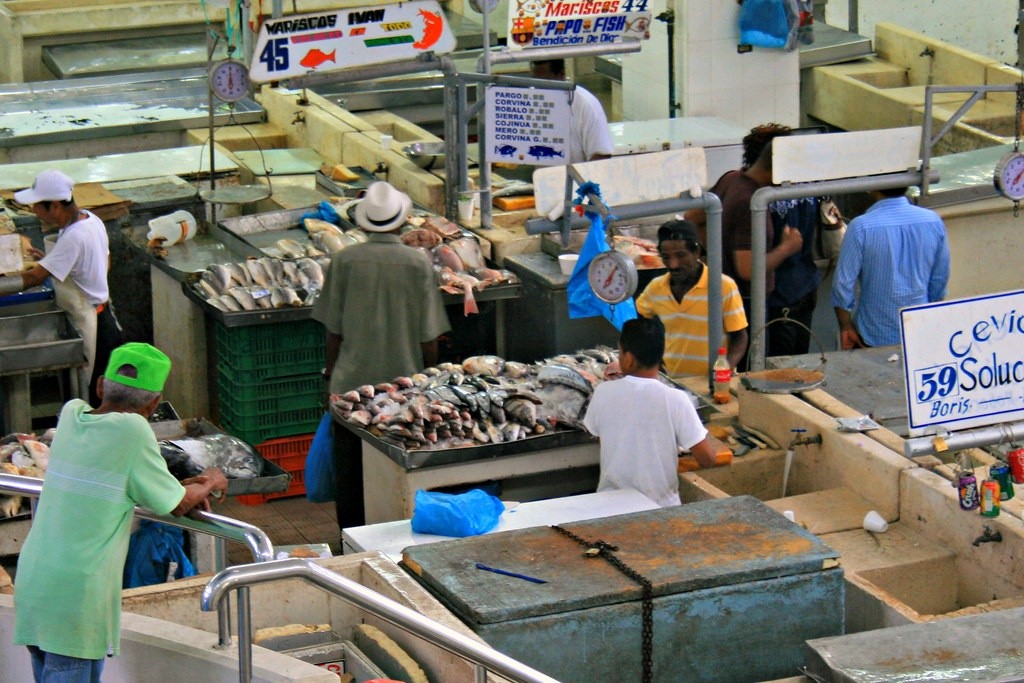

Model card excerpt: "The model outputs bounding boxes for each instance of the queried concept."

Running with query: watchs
[320,366,330,381]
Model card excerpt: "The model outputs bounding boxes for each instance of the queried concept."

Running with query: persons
[12,343,229,683]
[583,314,715,509]
[0,169,124,410]
[531,59,613,169]
[683,122,833,372]
[635,221,750,379]
[829,170,952,351]
[310,181,451,546]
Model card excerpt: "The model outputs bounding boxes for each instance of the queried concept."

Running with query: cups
[457,199,475,220]
[863,510,888,533]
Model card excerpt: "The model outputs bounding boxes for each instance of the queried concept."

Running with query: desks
[362,440,602,524]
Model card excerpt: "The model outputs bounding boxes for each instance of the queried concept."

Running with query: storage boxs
[209,324,330,506]
[397,492,848,683]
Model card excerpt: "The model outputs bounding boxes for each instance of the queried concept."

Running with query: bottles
[712,348,731,404]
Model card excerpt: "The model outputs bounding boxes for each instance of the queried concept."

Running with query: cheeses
[331,164,362,182]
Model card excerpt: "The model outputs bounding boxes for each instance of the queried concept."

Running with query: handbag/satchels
[303,411,336,504]
[124,522,196,588]
[411,488,506,537]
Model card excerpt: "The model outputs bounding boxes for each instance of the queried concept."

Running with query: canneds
[958,444,1024,518]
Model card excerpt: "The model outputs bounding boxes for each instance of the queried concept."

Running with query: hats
[15,168,76,205]
[354,181,413,231]
[105,343,172,391]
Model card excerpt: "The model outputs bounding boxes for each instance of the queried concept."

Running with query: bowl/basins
[558,254,579,275]
[402,142,445,170]
